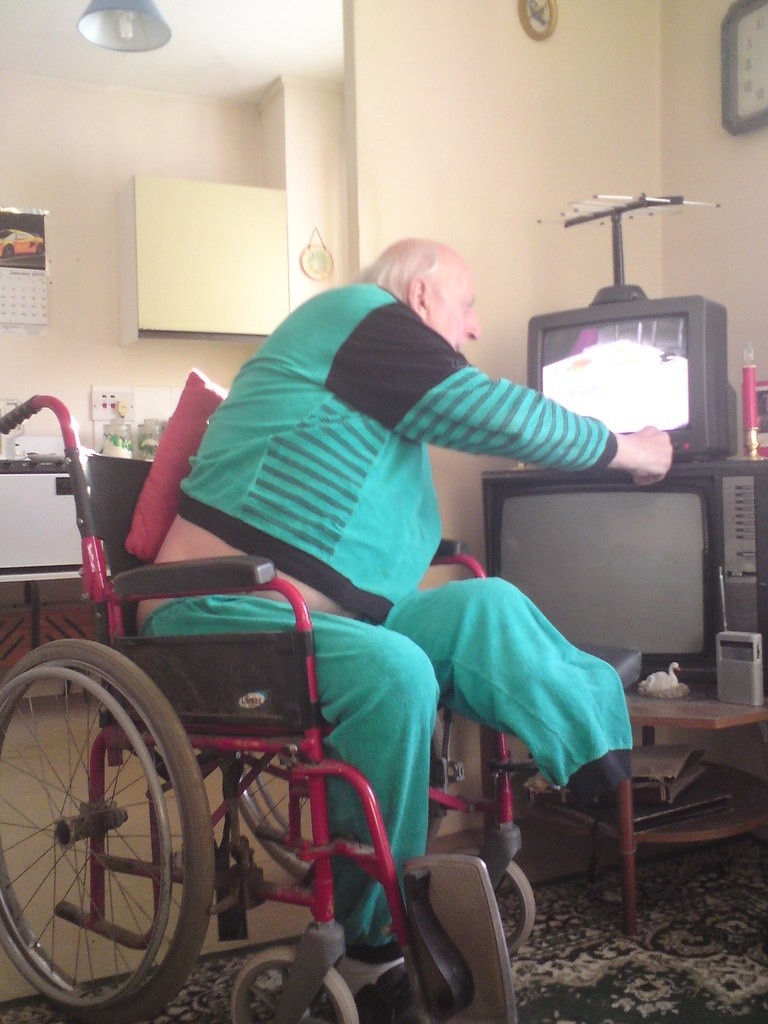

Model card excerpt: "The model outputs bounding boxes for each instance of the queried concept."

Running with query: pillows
[124,365,231,559]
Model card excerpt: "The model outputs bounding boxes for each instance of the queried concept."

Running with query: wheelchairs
[0,394,539,1023]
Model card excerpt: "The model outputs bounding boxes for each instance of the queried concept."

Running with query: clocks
[518,0,558,40]
[721,0,768,137]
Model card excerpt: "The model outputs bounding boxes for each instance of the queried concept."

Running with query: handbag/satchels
[521,743,707,809]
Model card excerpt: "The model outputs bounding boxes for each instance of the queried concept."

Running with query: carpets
[0,827,768,1023]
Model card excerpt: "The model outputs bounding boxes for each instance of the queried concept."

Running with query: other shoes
[343,940,405,971]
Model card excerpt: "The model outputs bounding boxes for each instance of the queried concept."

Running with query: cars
[0,229,44,258]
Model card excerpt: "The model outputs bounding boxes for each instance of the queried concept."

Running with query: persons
[133,235,674,1024]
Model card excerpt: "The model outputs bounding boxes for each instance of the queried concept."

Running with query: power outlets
[91,388,136,420]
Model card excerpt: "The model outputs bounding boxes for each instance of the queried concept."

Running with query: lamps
[740,341,760,459]
[76,0,172,54]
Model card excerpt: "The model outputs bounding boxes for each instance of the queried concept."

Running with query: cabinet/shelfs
[120,172,291,346]
[0,459,83,652]
[481,690,768,933]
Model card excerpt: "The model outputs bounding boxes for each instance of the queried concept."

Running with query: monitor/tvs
[481,457,768,685]
[528,295,735,461]
